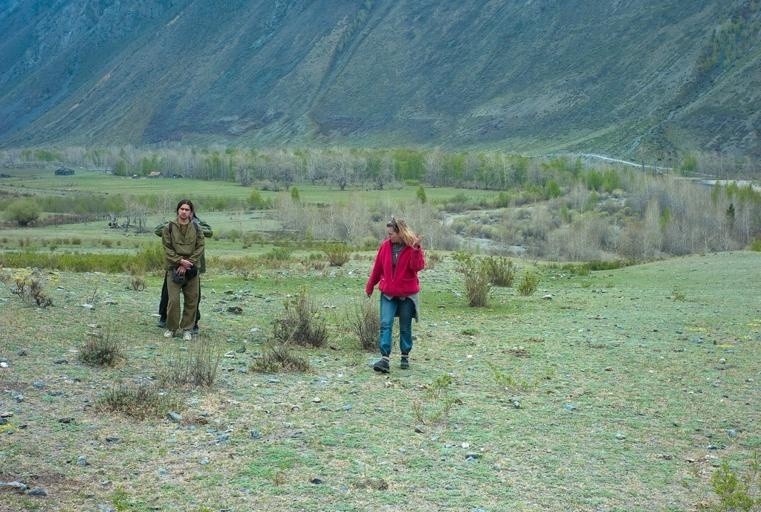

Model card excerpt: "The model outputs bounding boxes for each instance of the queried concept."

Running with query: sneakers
[192,324,199,334]
[182,330,193,341]
[157,320,166,327]
[373,359,390,373]
[163,328,176,338]
[400,360,409,370]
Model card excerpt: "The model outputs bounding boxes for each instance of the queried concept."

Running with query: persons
[154,199,213,341]
[365,219,424,373]
